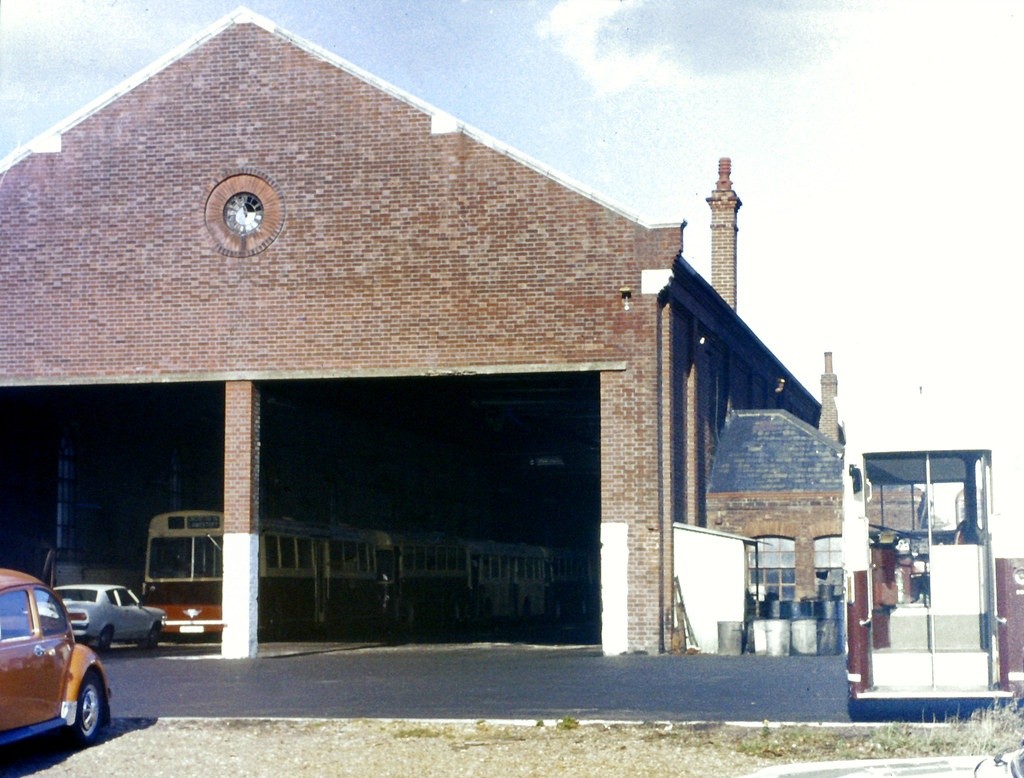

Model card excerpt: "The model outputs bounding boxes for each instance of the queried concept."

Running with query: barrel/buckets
[718,599,842,657]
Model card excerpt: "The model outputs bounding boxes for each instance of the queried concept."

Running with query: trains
[142,511,597,633]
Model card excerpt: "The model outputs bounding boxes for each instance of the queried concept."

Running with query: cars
[47,584,168,650]
[0,568,112,744]
